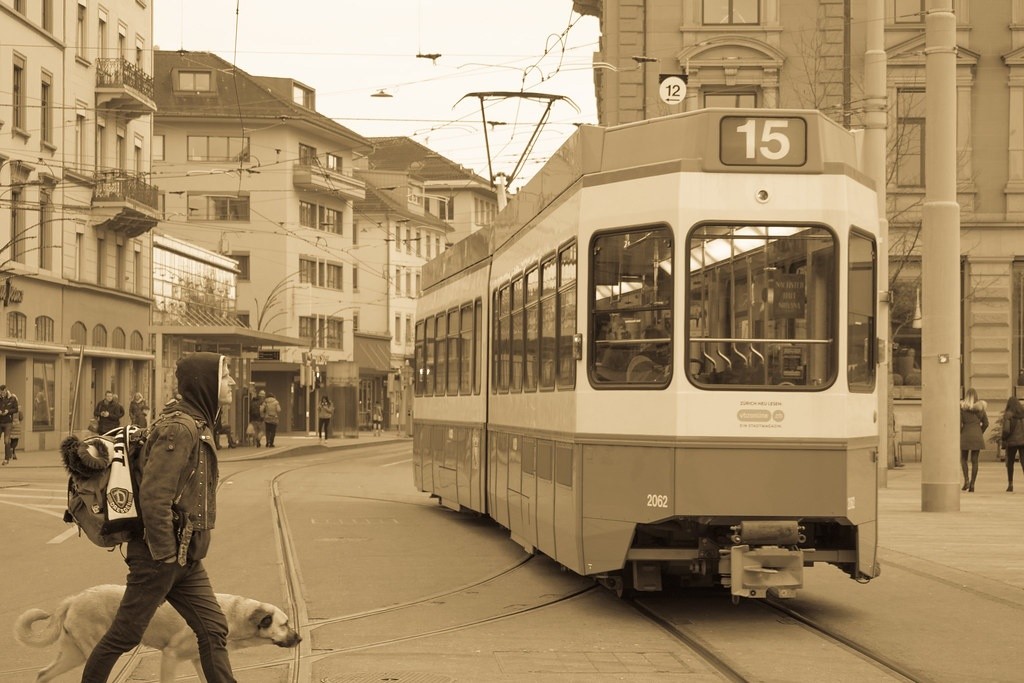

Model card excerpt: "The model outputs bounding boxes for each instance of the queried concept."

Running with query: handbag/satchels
[88,415,100,433]
[265,410,279,419]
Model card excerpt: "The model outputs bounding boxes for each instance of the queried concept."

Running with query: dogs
[16,583,304,683]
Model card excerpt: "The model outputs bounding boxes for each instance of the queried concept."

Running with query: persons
[249,389,266,448]
[317,395,334,444]
[81,351,238,683]
[1001,396,1024,492]
[112,394,125,427]
[34,392,48,424]
[595,313,669,371]
[907,347,920,369]
[259,393,281,448]
[128,393,150,428]
[372,400,383,437]
[94,390,121,436]
[10,394,24,460]
[0,385,19,466]
[960,388,990,492]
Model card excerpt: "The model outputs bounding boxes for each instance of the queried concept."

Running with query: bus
[409,105,893,611]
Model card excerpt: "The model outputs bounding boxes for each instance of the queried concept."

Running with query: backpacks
[249,398,264,417]
[67,411,201,548]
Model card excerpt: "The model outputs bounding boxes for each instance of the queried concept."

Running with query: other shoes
[266,442,275,447]
[1006,486,1013,491]
[256,438,261,447]
[229,443,236,448]
[217,445,222,450]
[2,460,9,465]
[10,453,17,459]
[962,484,975,492]
